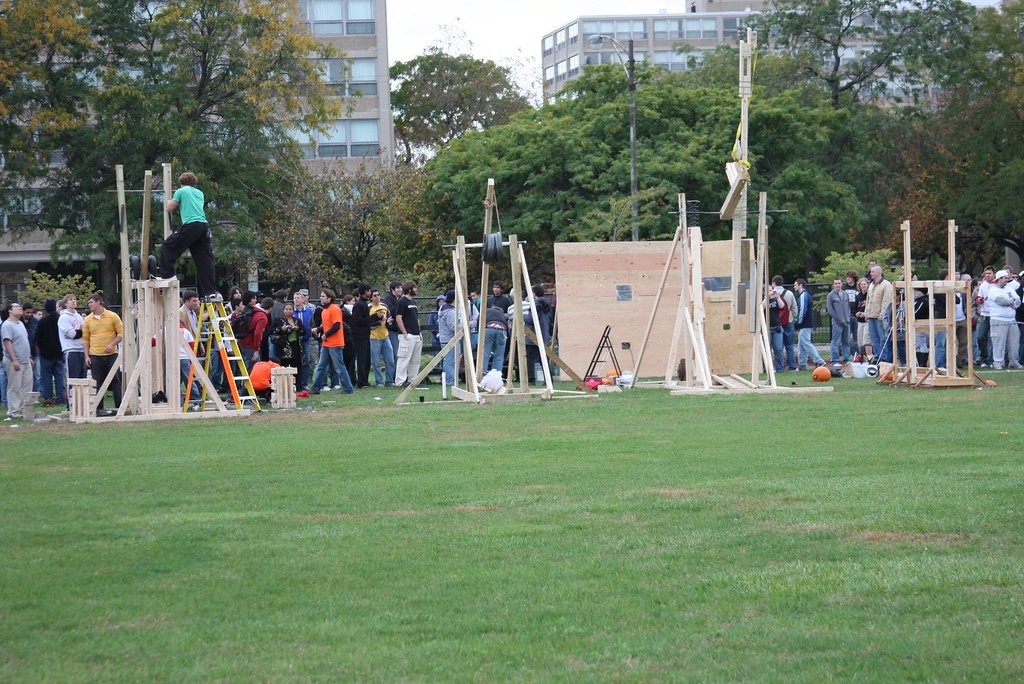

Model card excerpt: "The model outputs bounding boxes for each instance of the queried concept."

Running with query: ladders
[583,325,621,382]
[182,298,261,413]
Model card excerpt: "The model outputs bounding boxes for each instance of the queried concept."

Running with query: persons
[826,259,1024,369]
[0,294,123,418]
[179,281,423,403]
[152,321,201,412]
[763,275,827,374]
[156,171,217,298]
[427,281,554,385]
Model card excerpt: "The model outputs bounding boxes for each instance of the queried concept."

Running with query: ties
[190,309,193,316]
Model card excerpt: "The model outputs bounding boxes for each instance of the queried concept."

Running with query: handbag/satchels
[269,333,287,345]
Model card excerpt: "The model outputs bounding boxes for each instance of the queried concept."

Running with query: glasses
[373,294,380,297]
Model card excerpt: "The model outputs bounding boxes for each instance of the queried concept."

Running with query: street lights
[586,33,643,239]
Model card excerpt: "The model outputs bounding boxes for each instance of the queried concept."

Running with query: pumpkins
[811,366,831,382]
[878,370,894,381]
[606,369,619,376]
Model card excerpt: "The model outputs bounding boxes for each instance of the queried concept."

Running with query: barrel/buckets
[850,362,869,378]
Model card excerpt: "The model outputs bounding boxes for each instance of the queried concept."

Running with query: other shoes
[205,291,215,298]
[42,398,65,406]
[296,374,556,394]
[981,363,987,367]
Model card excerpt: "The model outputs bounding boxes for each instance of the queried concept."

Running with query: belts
[486,326,506,330]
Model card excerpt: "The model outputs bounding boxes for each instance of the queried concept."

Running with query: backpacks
[778,289,789,327]
[232,305,257,339]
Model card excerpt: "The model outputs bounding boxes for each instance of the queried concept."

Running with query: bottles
[535,363,544,381]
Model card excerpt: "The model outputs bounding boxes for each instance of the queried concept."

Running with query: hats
[42,299,57,310]
[992,269,1008,282]
[299,289,308,295]
[446,290,455,300]
